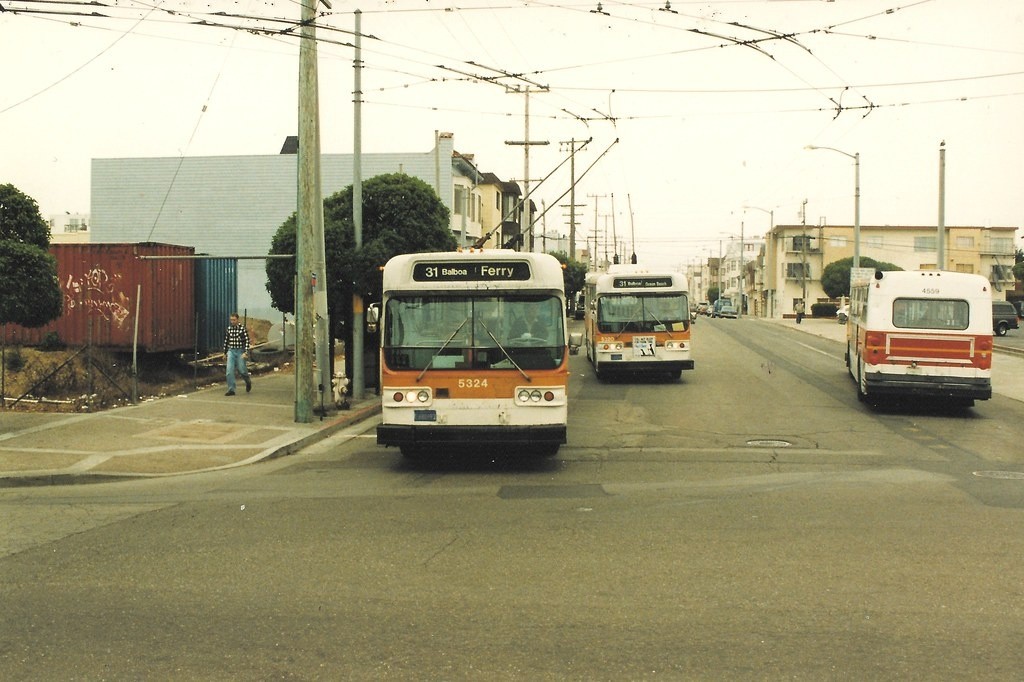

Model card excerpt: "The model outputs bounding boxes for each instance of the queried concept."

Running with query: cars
[696,299,737,319]
[992,301,1020,336]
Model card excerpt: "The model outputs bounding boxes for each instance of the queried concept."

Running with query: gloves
[522,333,531,339]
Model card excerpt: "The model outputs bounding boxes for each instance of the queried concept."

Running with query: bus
[584,263,696,383]
[367,249,583,465]
[837,270,994,419]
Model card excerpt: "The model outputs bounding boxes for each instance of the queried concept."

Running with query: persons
[793,301,804,323]
[221,313,251,395]
[507,303,549,343]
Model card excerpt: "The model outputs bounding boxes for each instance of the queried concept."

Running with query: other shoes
[246,379,251,392]
[225,392,235,396]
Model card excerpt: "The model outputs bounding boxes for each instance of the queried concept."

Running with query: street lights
[804,145,860,270]
